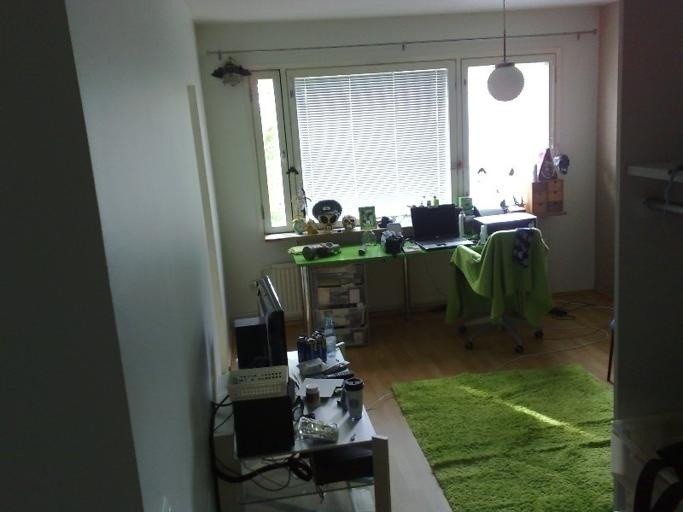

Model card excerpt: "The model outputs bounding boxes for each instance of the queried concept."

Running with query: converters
[549,307,567,316]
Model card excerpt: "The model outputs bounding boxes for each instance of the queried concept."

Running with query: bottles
[457,209,465,238]
[342,376,364,420]
[323,311,336,357]
[479,225,487,245]
[305,383,319,409]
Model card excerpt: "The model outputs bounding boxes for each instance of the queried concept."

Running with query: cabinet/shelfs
[607,0,682,510]
[525,179,564,215]
[309,262,372,349]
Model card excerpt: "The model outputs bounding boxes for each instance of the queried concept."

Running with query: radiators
[262,262,302,321]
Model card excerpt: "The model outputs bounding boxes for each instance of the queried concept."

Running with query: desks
[286,237,480,340]
[211,344,391,510]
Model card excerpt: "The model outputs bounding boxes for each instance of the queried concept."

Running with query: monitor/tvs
[234,275,289,370]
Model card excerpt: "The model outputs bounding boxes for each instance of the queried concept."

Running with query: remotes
[323,360,351,375]
[324,371,354,379]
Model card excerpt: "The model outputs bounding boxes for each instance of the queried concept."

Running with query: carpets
[389,361,616,512]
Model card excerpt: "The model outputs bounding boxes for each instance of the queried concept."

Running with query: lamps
[211,56,250,87]
[485,1,525,102]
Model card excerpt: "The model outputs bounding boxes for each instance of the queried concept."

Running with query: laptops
[411,204,476,250]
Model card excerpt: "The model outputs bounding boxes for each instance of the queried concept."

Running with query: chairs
[442,226,551,355]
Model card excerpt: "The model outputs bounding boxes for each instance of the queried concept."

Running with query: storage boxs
[608,409,682,509]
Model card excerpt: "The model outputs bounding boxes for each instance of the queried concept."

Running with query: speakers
[232,377,296,460]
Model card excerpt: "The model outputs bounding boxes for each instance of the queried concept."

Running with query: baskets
[226,365,289,401]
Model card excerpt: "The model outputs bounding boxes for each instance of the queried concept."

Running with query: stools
[607,320,616,383]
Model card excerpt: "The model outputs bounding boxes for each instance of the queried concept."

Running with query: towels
[512,226,533,269]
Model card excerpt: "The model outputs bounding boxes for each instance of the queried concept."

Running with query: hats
[313,200,342,219]
